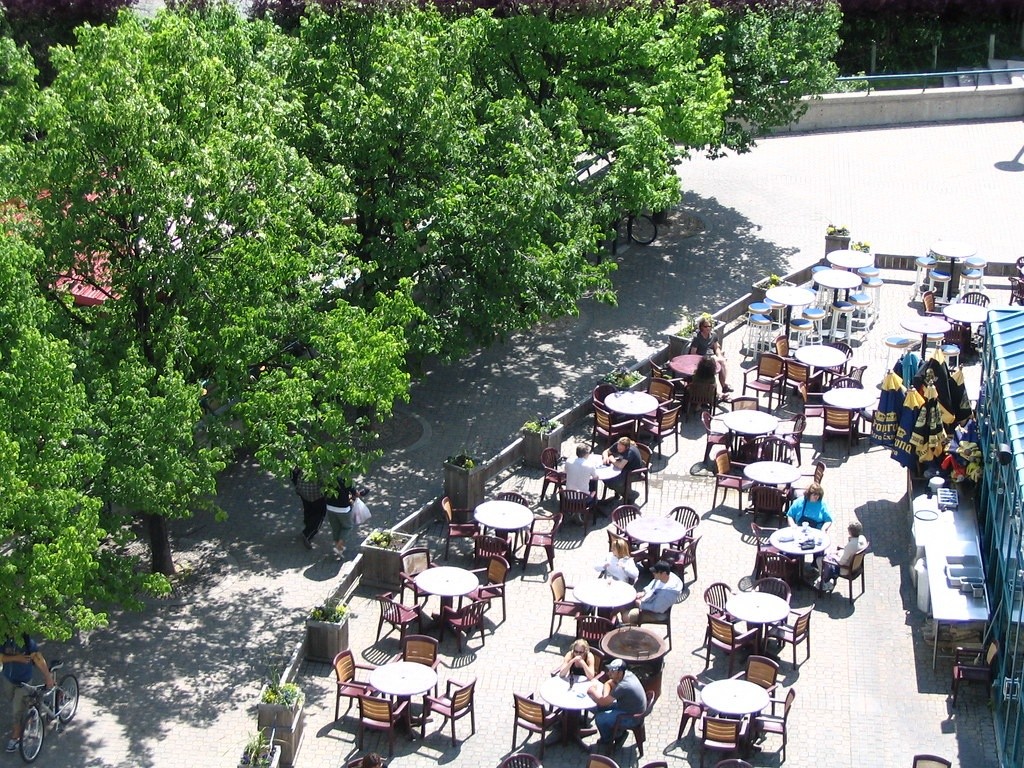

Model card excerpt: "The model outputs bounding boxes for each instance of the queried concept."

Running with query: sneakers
[6,737,21,753]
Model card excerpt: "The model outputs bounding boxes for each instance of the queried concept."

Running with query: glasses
[573,650,586,654]
[703,326,711,328]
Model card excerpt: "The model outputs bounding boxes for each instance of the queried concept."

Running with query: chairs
[332,244,1024,768]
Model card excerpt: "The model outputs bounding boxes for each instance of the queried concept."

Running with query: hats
[649,560,670,573]
[604,658,627,672]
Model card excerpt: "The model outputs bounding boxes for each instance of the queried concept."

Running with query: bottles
[570,671,574,688]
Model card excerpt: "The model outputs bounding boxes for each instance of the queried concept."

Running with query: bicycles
[19,660,79,763]
[601,187,658,245]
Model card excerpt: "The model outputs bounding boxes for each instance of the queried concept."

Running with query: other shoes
[826,581,834,590]
[301,533,313,551]
[615,730,629,751]
[597,735,614,744]
[814,582,828,590]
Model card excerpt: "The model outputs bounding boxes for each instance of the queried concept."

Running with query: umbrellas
[870,338,983,497]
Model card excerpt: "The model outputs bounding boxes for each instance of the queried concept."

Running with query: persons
[814,519,868,591]
[321,452,363,559]
[559,638,595,727]
[689,317,735,393]
[0,612,57,754]
[621,559,685,631]
[594,537,639,625]
[564,443,599,526]
[681,355,716,413]
[586,658,648,752]
[289,439,330,551]
[786,482,833,570]
[601,436,643,506]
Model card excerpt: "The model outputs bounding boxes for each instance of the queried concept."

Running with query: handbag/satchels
[351,497,372,526]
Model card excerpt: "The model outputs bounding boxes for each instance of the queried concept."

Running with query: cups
[802,521,809,533]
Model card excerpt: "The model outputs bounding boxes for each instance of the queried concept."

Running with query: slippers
[332,548,344,558]
[339,545,346,554]
